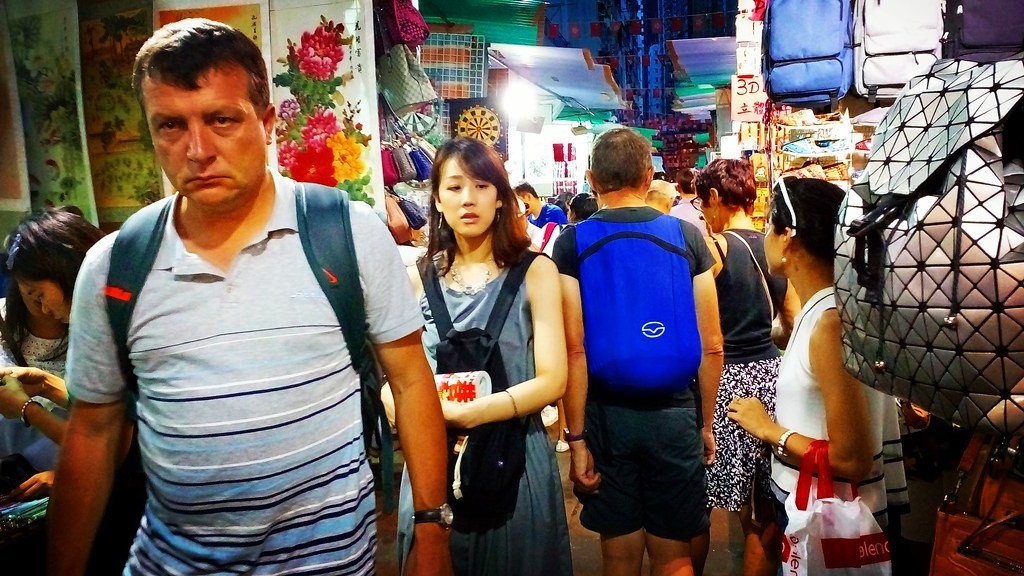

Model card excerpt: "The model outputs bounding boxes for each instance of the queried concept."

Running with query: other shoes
[779,162,850,186]
[778,135,853,157]
[775,107,834,130]
[852,170,865,180]
[856,133,873,154]
[555,440,570,452]
[850,106,891,127]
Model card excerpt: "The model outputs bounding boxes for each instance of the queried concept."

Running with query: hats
[648,180,676,200]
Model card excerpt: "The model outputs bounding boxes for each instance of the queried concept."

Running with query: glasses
[773,176,800,227]
[690,195,702,210]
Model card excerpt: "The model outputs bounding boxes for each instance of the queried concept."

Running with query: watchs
[777,431,798,456]
[416,504,454,530]
[21,400,44,427]
[562,427,589,442]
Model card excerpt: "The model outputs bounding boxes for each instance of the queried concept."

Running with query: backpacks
[417,249,546,535]
[947,0,1024,63]
[853,1,946,101]
[760,1,852,112]
[834,50,1024,429]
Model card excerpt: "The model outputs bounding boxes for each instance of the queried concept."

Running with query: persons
[387,126,975,576]
[0,202,148,576]
[48,18,456,576]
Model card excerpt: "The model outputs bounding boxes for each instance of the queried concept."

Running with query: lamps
[571,118,587,136]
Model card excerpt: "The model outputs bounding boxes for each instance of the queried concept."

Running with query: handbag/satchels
[376,96,447,241]
[374,0,429,48]
[375,43,439,115]
[782,440,892,575]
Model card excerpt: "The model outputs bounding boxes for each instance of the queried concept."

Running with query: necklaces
[448,258,494,296]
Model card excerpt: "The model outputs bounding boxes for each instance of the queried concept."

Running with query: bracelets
[504,389,519,418]
